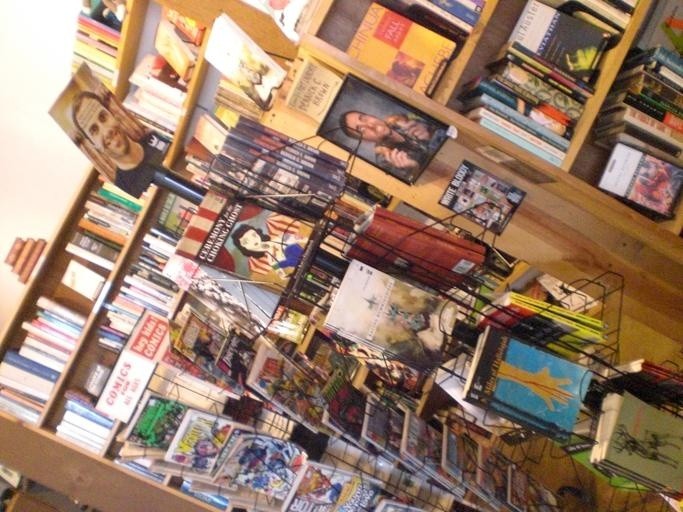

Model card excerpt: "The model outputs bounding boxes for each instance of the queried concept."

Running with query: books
[1,1,683,511]
